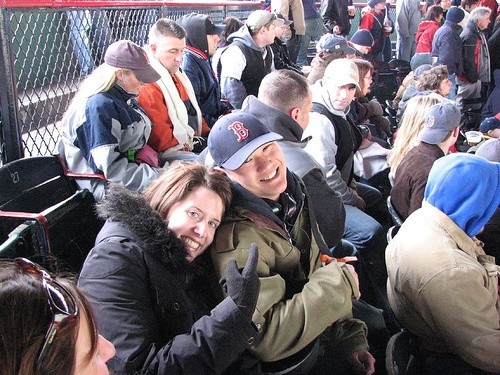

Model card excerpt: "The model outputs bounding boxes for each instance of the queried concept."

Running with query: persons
[76,159,262,375]
[299,58,390,317]
[174,13,237,129]
[0,257,117,375]
[209,113,376,375]
[132,18,211,162]
[385,153,500,375]
[202,70,388,358]
[61,40,169,215]
[211,0,500,264]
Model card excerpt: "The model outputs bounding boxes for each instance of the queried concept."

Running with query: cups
[385,22,391,32]
[465,130,483,147]
[420,2,426,10]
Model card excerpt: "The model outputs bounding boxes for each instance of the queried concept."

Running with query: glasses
[364,76,373,81]
[14,257,78,375]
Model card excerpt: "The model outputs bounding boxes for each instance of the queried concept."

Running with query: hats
[104,40,162,83]
[271,12,294,26]
[446,6,465,23]
[320,35,356,56]
[205,18,226,35]
[413,63,433,80]
[325,57,361,91]
[475,138,500,162]
[367,0,385,8]
[418,102,461,144]
[208,113,284,170]
[479,117,500,133]
[247,10,285,29]
[349,29,374,47]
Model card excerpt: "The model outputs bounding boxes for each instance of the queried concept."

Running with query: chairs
[386,333,430,375]
[0,153,108,273]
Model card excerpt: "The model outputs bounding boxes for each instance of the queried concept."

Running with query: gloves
[219,242,262,323]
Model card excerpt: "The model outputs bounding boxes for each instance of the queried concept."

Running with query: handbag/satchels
[135,145,159,169]
